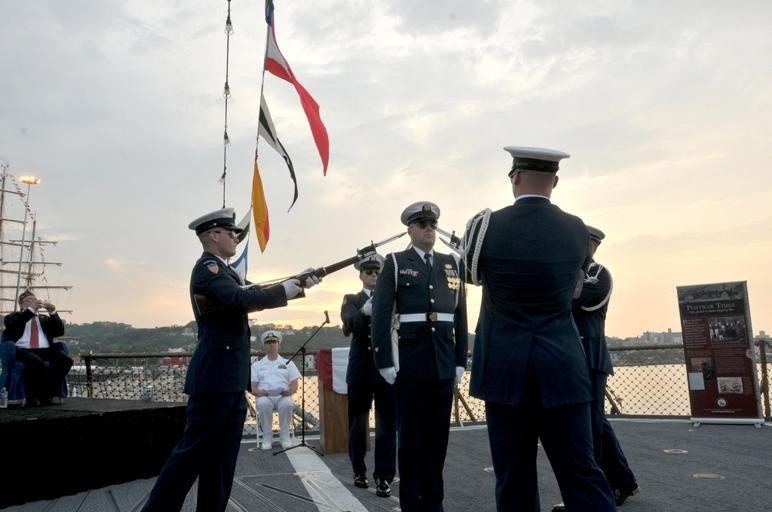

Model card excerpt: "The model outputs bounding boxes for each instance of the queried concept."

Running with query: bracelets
[264,391,266,396]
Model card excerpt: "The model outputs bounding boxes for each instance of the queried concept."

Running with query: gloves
[378,367,397,385]
[280,279,302,300]
[363,295,373,316]
[454,366,466,383]
[296,266,322,289]
[264,386,284,397]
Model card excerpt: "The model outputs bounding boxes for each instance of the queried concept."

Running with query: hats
[261,330,283,346]
[354,253,385,270]
[18,291,34,302]
[401,201,440,226]
[584,224,605,246]
[188,206,243,234]
[503,145,570,177]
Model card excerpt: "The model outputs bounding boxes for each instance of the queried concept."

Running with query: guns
[240,232,406,300]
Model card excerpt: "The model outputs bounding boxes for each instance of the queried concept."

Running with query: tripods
[273,320,328,456]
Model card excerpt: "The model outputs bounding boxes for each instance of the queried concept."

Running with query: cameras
[40,299,50,308]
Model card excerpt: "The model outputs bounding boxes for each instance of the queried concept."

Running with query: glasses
[414,223,437,229]
[362,271,380,276]
[207,229,235,239]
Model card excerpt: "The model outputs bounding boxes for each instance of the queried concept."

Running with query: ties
[30,315,39,348]
[370,289,375,296]
[424,254,433,272]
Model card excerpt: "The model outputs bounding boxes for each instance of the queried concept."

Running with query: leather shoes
[374,477,392,497]
[552,500,565,512]
[261,440,273,450]
[279,440,292,449]
[615,481,640,505]
[354,471,370,489]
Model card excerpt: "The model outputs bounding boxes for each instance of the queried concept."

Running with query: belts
[399,312,455,324]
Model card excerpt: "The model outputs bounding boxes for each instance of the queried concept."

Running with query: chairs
[0,324,69,406]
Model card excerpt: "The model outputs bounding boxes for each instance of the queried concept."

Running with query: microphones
[324,310,329,323]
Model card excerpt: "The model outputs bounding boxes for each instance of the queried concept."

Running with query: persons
[708,320,745,340]
[371,200,468,511]
[139,209,320,509]
[460,146,622,510]
[693,360,712,385]
[682,285,743,301]
[340,253,396,496]
[552,226,640,511]
[6,291,74,406]
[251,331,302,450]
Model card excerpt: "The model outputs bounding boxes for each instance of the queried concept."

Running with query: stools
[252,398,294,451]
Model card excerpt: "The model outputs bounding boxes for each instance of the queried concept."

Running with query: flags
[259,85,298,212]
[266,0,330,177]
[252,159,271,253]
[227,207,252,284]
